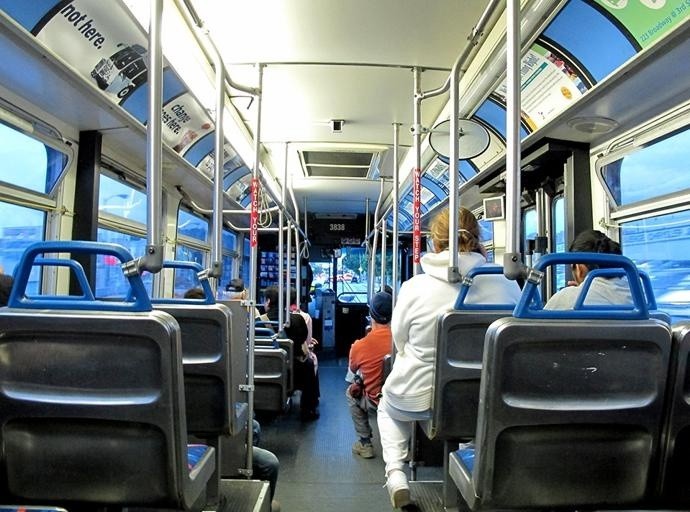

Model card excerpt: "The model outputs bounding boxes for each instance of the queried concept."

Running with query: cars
[323,272,359,284]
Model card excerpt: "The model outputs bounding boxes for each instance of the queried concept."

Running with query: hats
[371,291,393,323]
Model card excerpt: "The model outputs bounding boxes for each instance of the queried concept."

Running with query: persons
[222,277,260,324]
[241,418,283,511]
[345,292,394,459]
[183,289,209,300]
[254,285,321,427]
[609,241,635,302]
[375,205,523,508]
[541,230,634,312]
[283,286,321,407]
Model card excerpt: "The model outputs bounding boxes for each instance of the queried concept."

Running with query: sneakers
[299,408,320,423]
[386,471,411,507]
[269,499,281,511]
[351,440,373,458]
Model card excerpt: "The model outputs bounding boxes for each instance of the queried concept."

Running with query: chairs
[383,251,689,511]
[0,241,297,512]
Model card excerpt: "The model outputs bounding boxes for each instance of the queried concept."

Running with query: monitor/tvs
[482,195,505,221]
